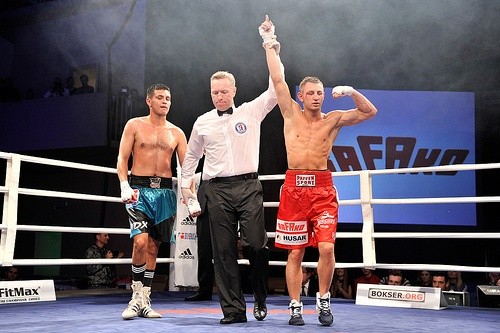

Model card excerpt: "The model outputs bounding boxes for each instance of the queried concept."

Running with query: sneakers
[138,287,160,317]
[287,299,305,325]
[316,292,333,326]
[122,280,144,319]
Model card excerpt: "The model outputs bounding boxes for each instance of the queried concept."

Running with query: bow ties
[217,107,233,116]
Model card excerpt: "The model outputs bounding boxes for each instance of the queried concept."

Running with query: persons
[259,16,379,325]
[46,74,94,98]
[118,84,200,318]
[114,86,147,120]
[184,218,500,306]
[86,233,123,290]
[180,35,284,324]
[0,267,17,281]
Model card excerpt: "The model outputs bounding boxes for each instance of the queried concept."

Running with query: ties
[301,286,306,296]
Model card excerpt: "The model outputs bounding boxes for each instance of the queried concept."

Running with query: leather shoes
[253,294,267,320]
[220,315,247,324]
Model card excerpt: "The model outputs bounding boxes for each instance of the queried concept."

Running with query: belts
[209,172,258,183]
